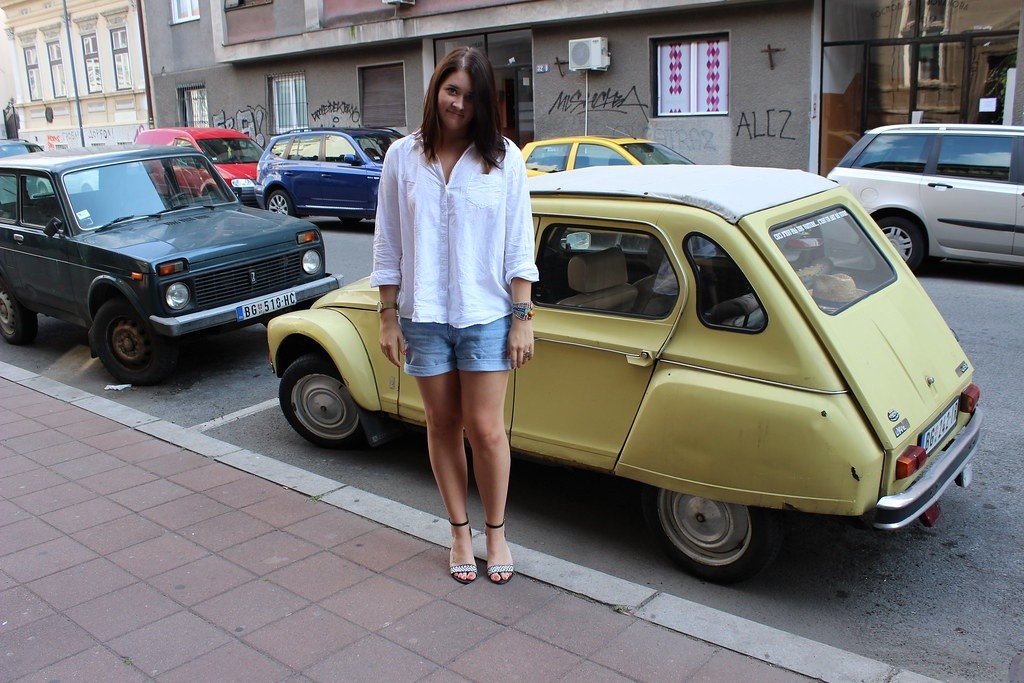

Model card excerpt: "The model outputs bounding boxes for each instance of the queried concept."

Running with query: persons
[367,46,540,589]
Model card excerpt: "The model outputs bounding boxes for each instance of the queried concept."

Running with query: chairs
[557,246,836,331]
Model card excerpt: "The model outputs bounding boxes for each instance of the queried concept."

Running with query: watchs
[376,301,399,314]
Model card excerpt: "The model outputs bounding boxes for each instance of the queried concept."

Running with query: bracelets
[511,302,535,321]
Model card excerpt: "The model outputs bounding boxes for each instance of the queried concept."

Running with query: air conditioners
[569,36,610,71]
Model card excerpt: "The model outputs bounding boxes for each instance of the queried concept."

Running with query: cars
[520,136,696,178]
[265,166,986,583]
[0,138,44,158]
[254,125,409,227]
[134,126,268,209]
[0,145,340,388]
[826,127,1024,278]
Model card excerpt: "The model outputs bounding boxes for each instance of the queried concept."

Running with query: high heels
[449,512,478,584]
[484,519,514,585]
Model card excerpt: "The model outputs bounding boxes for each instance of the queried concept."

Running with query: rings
[523,350,530,358]
